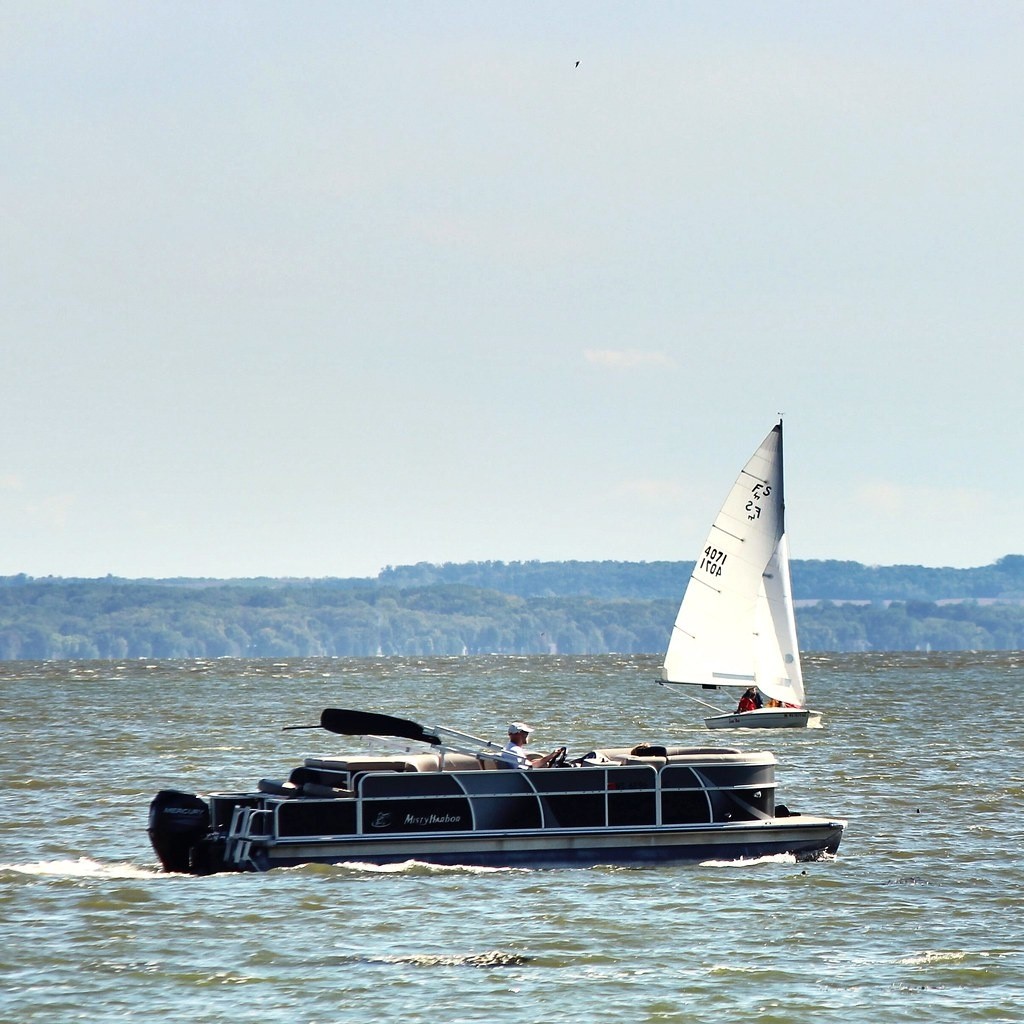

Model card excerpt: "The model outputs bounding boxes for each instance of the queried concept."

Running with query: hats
[508,722,532,735]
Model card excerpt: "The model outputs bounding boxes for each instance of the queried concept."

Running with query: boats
[143,705,847,880]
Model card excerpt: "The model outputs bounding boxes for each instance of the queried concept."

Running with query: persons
[737,688,763,713]
[502,722,561,770]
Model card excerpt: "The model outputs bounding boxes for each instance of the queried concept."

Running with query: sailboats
[653,417,823,729]
